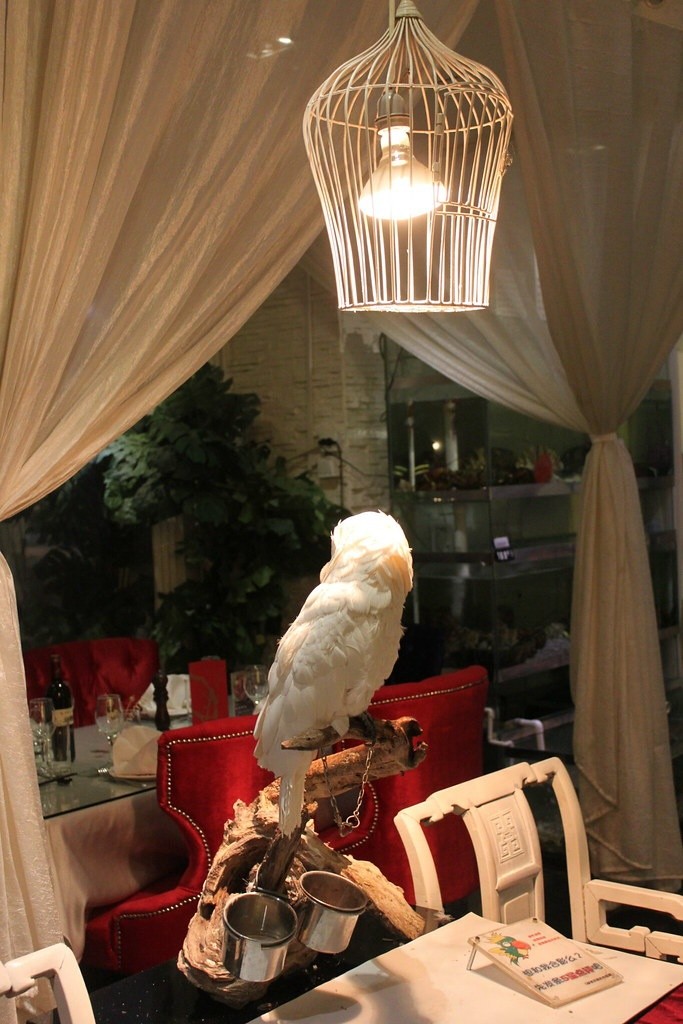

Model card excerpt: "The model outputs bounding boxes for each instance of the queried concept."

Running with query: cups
[296,870,368,954]
[221,892,299,982]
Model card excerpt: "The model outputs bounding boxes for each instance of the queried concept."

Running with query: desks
[27,905,683,1024]
[31,698,252,962]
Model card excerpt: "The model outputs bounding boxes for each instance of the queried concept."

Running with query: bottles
[45,653,75,762]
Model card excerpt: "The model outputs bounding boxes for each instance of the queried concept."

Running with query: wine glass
[95,693,124,773]
[243,664,268,708]
[29,698,57,779]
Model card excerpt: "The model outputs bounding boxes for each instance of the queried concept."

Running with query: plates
[107,763,158,783]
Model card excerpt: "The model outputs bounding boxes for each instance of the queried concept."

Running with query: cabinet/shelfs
[382,334,683,753]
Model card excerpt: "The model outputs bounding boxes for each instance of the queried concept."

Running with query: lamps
[302,0,514,312]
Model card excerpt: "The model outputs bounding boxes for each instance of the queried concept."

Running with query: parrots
[252,508,413,842]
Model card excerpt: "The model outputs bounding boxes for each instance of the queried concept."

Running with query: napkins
[109,724,162,781]
[137,674,193,717]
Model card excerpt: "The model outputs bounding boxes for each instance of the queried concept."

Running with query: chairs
[393,756,683,966]
[25,635,163,732]
[82,662,490,976]
